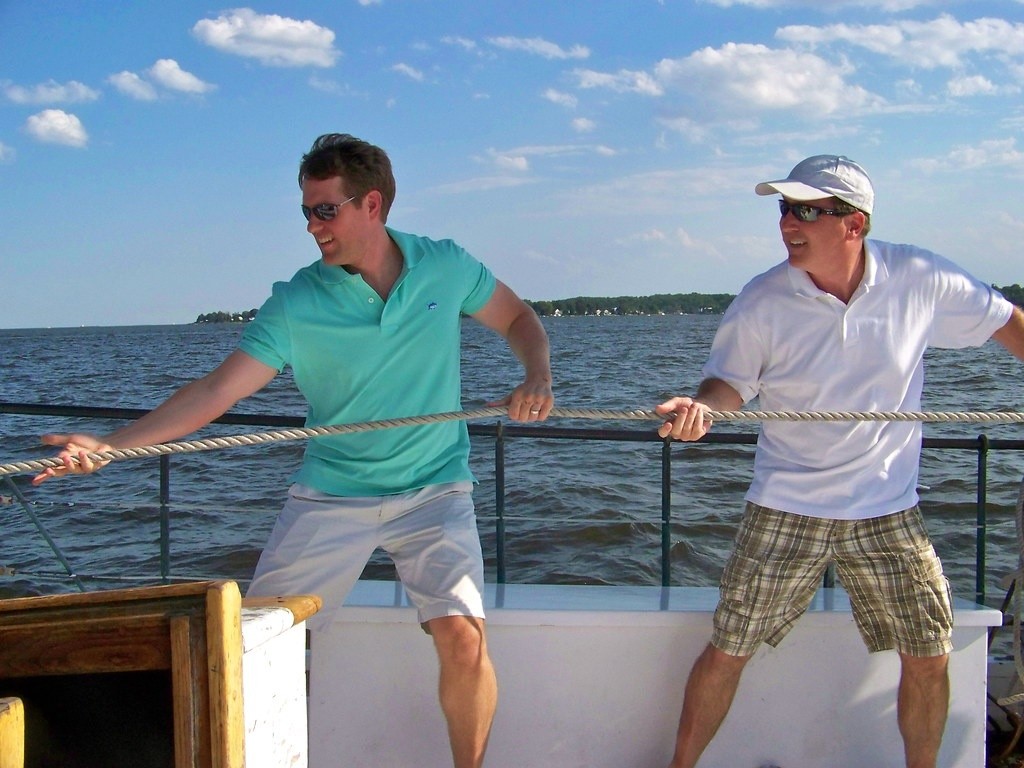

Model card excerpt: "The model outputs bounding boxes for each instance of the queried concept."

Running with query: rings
[529,408,540,415]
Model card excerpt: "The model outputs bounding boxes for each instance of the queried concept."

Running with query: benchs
[304,579,1002,768]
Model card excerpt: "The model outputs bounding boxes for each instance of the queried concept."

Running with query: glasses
[779,198,856,223]
[301,194,367,221]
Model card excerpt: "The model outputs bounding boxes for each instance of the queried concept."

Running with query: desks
[0,579,322,768]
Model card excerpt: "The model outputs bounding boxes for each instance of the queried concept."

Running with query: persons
[32,135,555,766]
[655,153,1024,768]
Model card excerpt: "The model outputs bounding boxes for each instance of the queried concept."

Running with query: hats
[755,154,875,215]
